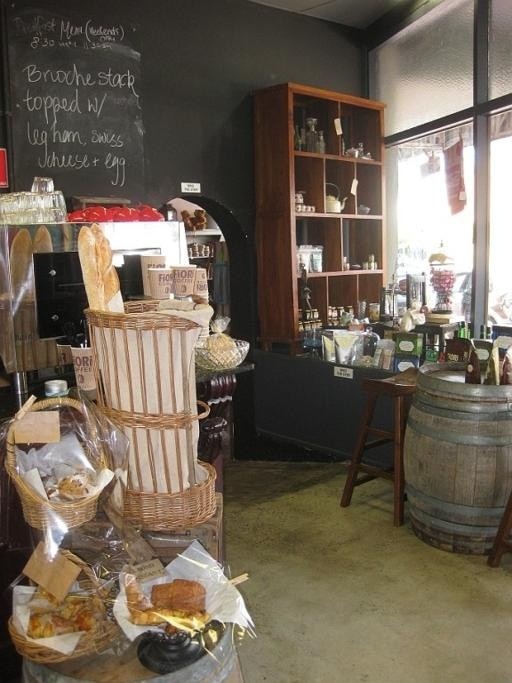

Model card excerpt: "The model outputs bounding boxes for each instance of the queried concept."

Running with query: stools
[340,367,419,527]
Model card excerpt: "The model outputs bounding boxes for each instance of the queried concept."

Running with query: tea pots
[326,182,348,214]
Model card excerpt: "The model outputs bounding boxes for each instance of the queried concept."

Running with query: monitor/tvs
[32,247,161,344]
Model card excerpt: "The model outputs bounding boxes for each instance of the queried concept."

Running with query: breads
[44,474,84,500]
[26,584,109,639]
[76,223,125,314]
[125,578,209,632]
[8,225,53,299]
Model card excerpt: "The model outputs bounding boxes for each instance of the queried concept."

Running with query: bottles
[480,325,491,339]
[360,326,380,358]
[297,308,323,332]
[327,305,355,327]
[295,124,326,154]
[44,379,68,409]
[428,239,457,314]
[460,320,471,339]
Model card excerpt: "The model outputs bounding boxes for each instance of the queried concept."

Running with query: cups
[70,346,96,392]
[363,253,378,270]
[169,264,197,300]
[148,268,174,300]
[0,177,67,224]
[294,194,316,213]
[193,268,209,296]
[140,254,166,297]
[369,303,380,323]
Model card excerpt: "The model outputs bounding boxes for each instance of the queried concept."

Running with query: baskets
[104,560,222,642]
[83,300,217,532]
[4,397,108,530]
[7,549,121,665]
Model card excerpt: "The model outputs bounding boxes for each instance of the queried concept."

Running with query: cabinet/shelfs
[251,81,388,357]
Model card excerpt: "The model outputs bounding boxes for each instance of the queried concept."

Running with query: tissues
[394,309,427,357]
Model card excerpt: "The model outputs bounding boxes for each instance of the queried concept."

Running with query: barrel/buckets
[402,362,512,556]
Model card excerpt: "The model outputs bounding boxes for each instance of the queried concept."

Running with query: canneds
[298,305,355,333]
[343,252,377,271]
